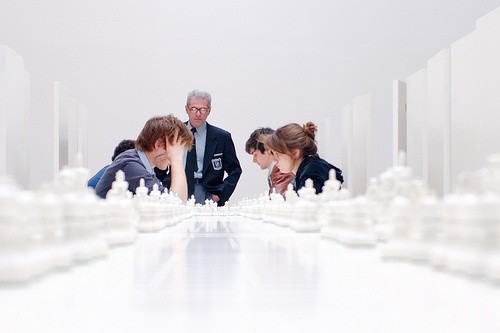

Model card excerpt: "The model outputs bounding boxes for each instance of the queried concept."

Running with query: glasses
[187,106,209,114]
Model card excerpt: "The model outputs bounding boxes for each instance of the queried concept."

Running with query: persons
[155,89,242,206]
[245,127,297,200]
[87,139,135,189]
[95,113,193,204]
[262,120,344,195]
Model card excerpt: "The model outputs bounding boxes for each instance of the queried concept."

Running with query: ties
[185,129,198,199]
